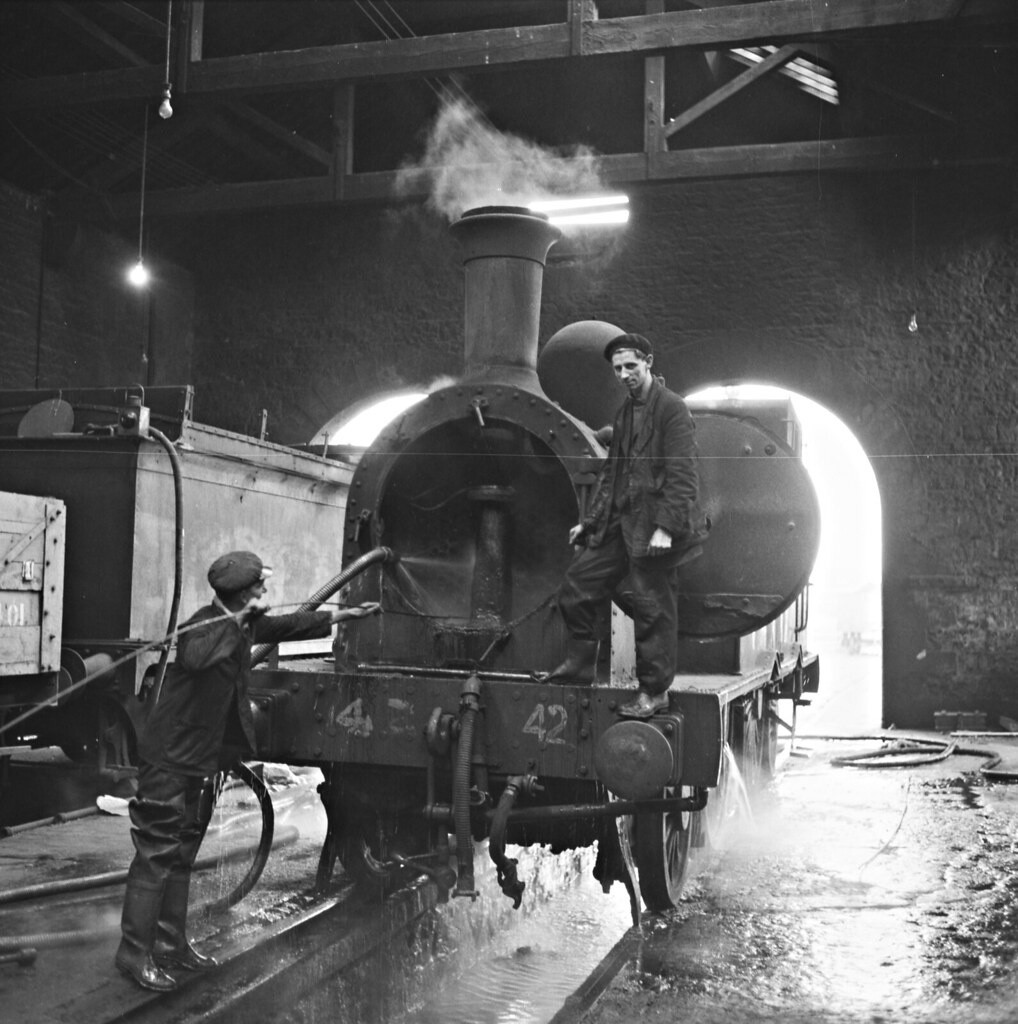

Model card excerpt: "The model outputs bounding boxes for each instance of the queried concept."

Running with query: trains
[0,380,347,790]
[130,200,819,917]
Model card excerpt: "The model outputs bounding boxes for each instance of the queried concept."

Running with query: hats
[208,552,273,593]
[603,333,652,362]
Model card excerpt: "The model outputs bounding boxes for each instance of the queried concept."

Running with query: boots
[617,690,669,717]
[114,878,219,992]
[530,637,599,684]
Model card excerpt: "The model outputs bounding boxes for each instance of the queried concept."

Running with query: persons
[530,334,709,716]
[115,551,384,992]
